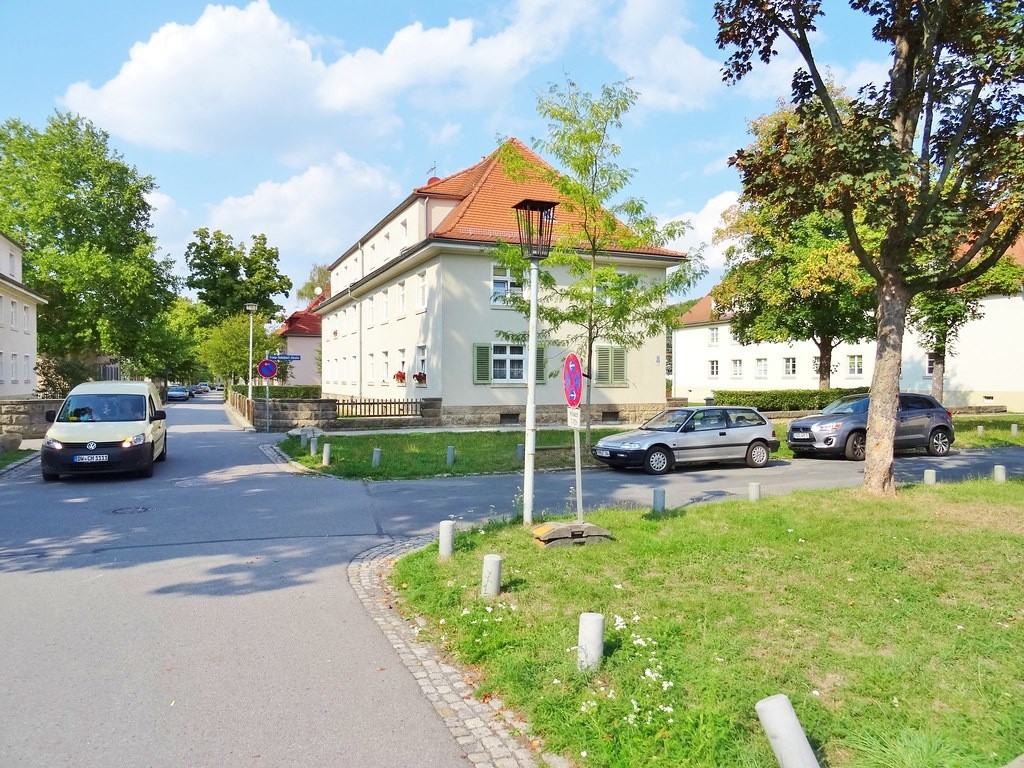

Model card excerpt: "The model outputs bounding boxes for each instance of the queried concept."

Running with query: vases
[420,379,426,383]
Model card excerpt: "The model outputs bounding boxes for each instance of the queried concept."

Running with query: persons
[79,403,103,421]
[122,401,142,420]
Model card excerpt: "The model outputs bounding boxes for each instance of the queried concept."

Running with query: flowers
[412,372,426,384]
[391,371,406,383]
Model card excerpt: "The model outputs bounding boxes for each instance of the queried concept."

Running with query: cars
[217,384,224,391]
[591,406,780,475]
[41,380,167,483]
[785,393,955,461]
[167,383,210,401]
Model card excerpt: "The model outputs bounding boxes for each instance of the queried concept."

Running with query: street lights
[511,199,561,526]
[244,303,260,399]
